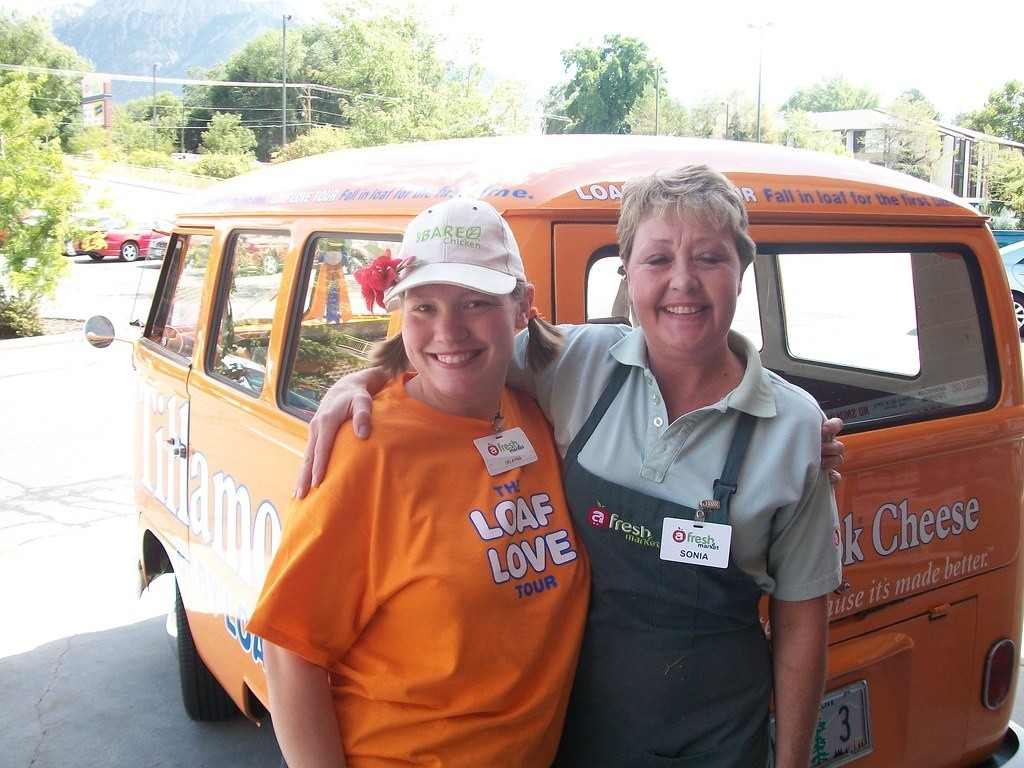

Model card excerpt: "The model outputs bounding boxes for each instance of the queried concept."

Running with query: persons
[292,163,842,768]
[245,199,846,768]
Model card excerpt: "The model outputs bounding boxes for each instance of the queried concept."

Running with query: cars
[0,209,112,257]
[74,217,165,263]
[998,240,1024,339]
[235,236,321,275]
[148,234,212,266]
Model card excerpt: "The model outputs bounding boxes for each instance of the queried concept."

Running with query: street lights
[721,103,729,139]
[650,67,664,136]
[747,21,774,143]
[153,63,162,152]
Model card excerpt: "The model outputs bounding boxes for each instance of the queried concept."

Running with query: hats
[380,197,529,313]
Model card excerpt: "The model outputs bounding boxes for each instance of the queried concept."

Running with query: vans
[84,135,1024,768]
